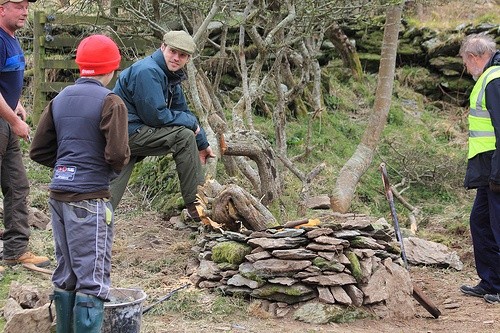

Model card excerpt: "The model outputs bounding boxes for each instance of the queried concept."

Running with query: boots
[48,287,75,333]
[73,292,104,333]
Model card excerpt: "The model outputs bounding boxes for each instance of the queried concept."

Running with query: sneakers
[484,293,500,304]
[460,282,497,297]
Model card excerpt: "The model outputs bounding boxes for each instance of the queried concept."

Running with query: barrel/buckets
[103,287,147,330]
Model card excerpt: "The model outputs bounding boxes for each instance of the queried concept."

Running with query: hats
[74,35,121,76]
[163,30,196,56]
[0,0,37,5]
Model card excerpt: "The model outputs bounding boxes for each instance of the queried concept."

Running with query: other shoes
[0,228,6,239]
[187,204,201,222]
[2,252,50,267]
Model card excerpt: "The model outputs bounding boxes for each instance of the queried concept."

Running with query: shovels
[381,163,441,318]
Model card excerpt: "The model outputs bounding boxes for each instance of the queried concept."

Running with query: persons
[29,34,130,333]
[460,32,500,305]
[110,31,215,224]
[0,0,49,267]
